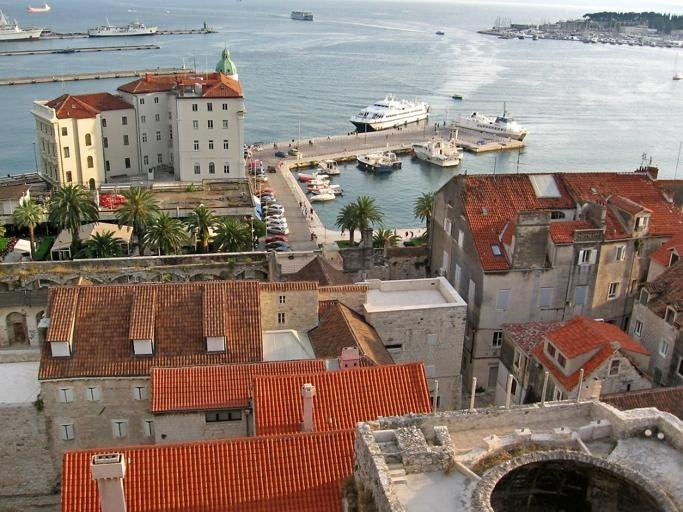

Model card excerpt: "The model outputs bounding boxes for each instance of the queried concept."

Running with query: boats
[436,31,444,36]
[411,123,463,168]
[455,100,528,141]
[356,154,394,175]
[672,73,682,80]
[318,158,341,175]
[451,95,462,100]
[382,142,401,170]
[25,2,50,13]
[297,171,342,203]
[348,93,430,131]
[495,30,682,50]
[0,9,43,41]
[86,15,157,38]
[289,11,313,21]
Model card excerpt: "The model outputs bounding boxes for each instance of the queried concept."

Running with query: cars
[267,246,292,252]
[243,150,276,174]
[255,187,289,236]
[264,235,288,242]
[264,240,289,248]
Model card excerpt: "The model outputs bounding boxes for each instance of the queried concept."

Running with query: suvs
[288,148,298,156]
[275,151,288,158]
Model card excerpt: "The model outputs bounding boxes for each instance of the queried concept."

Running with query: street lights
[31,140,38,176]
